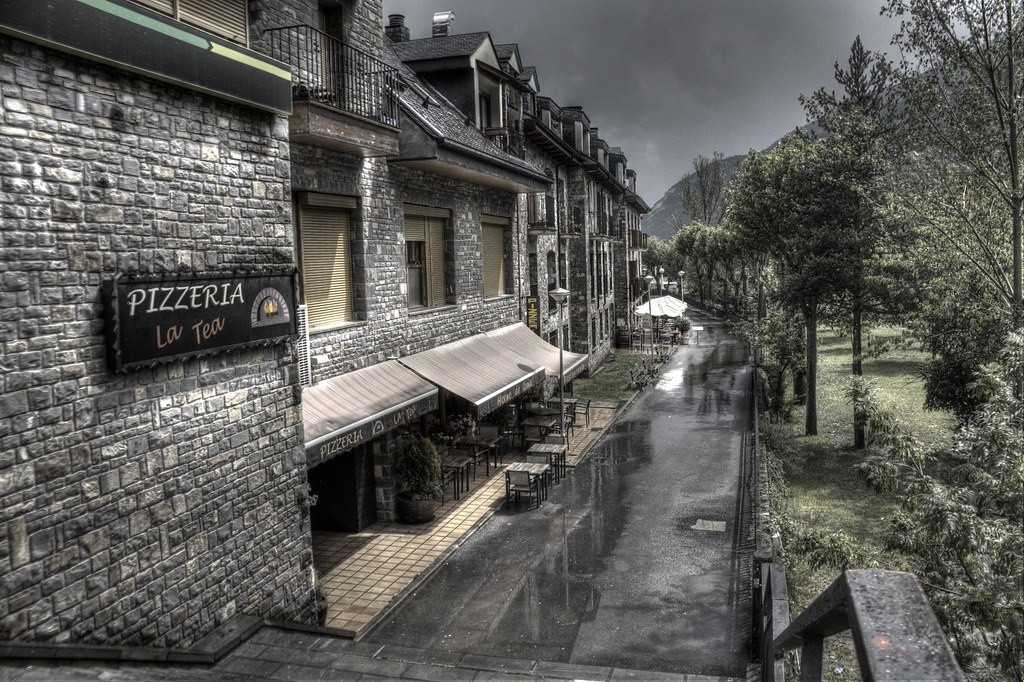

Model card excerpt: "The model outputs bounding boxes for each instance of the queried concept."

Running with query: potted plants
[393,435,440,525]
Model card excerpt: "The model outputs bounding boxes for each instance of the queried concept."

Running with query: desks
[455,435,500,476]
[525,407,560,432]
[521,418,557,448]
[438,455,472,505]
[503,462,550,510]
[527,442,568,483]
[546,397,578,423]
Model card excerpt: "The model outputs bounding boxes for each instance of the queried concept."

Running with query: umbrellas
[634,295,688,344]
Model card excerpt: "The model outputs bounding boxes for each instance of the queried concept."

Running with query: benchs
[643,357,659,376]
[630,365,650,389]
[655,345,669,363]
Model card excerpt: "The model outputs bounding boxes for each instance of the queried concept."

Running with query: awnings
[300,360,440,469]
[485,320,588,386]
[397,332,547,419]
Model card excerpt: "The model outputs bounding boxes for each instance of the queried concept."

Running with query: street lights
[658,267,665,297]
[677,270,685,301]
[642,275,658,367]
[549,287,572,440]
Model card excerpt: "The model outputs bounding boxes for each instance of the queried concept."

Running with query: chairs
[631,319,679,349]
[455,399,591,510]
